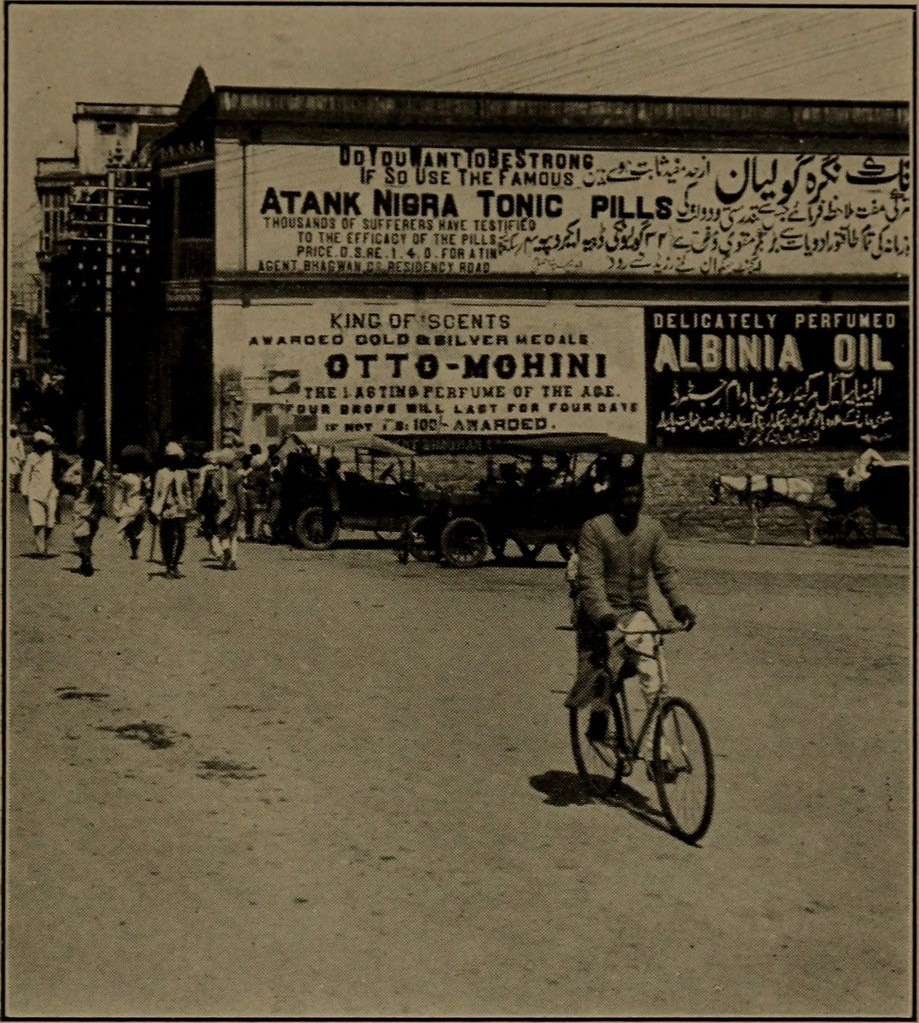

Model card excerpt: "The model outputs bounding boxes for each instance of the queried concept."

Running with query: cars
[286,428,442,552]
[422,432,647,569]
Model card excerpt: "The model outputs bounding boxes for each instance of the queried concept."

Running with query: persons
[496,453,608,491]
[151,443,196,577]
[566,467,696,743]
[66,437,109,575]
[197,443,339,569]
[9,424,24,491]
[115,445,152,560]
[22,431,59,556]
[831,434,882,480]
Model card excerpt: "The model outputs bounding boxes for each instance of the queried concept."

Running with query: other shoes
[646,760,677,784]
[585,709,610,741]
[32,540,240,579]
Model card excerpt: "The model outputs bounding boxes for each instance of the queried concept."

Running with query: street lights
[63,159,152,471]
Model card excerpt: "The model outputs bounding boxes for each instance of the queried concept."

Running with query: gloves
[673,604,698,632]
[599,613,618,632]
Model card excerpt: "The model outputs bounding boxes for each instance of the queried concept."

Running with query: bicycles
[565,601,716,844]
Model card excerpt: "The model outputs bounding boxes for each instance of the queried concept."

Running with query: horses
[707,471,819,547]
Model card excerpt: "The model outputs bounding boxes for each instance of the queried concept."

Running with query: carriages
[708,460,908,551]
